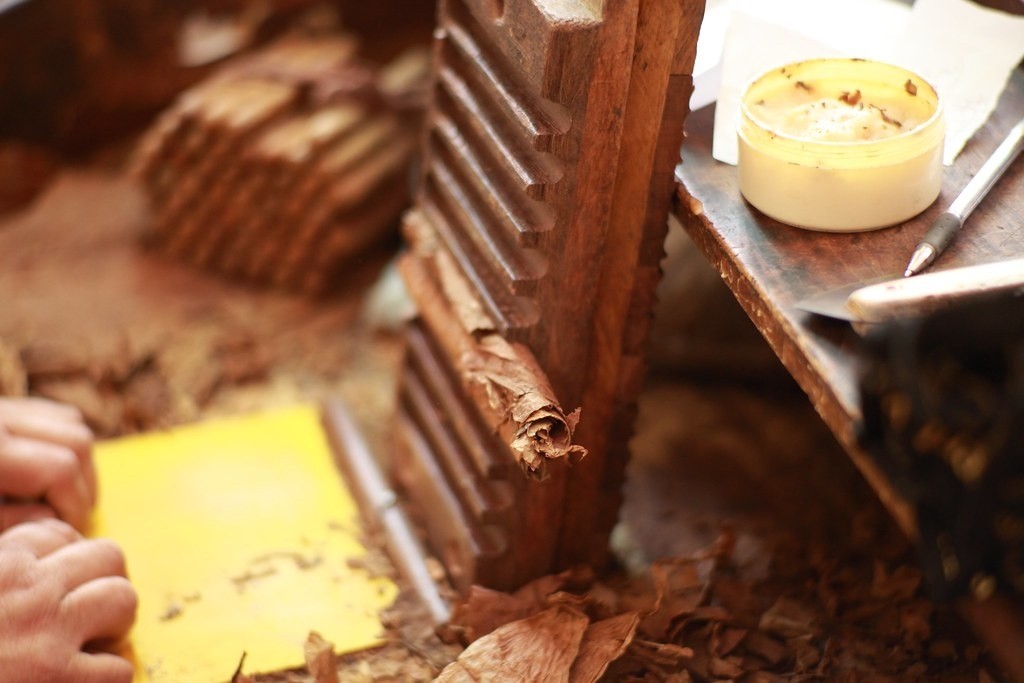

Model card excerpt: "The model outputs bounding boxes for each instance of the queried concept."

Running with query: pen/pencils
[904,120,1024,279]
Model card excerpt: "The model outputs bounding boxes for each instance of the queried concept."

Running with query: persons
[0,395,139,683]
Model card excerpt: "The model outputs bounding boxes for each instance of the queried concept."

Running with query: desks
[675,0,1024,683]
